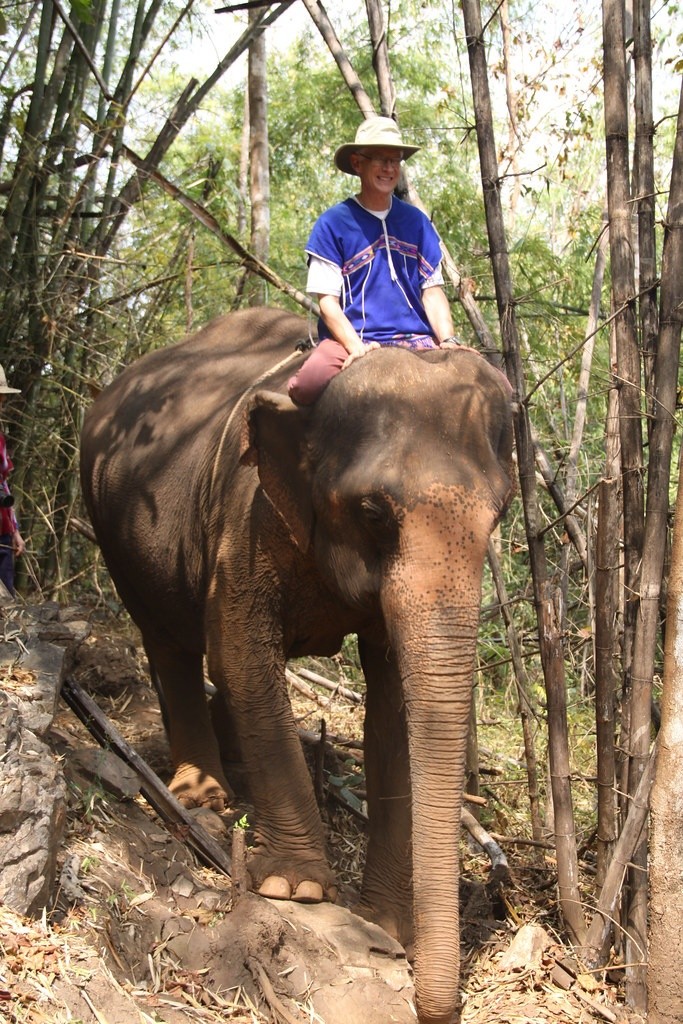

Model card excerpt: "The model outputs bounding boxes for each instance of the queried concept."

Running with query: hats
[0,364,22,394]
[334,117,422,176]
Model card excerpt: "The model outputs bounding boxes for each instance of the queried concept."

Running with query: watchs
[443,337,461,346]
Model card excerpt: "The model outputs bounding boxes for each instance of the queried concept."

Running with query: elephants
[75,302,523,1022]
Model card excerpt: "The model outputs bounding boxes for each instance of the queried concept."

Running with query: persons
[0,365,26,600]
[285,116,481,408]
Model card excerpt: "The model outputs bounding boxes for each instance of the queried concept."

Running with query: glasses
[359,153,405,168]
